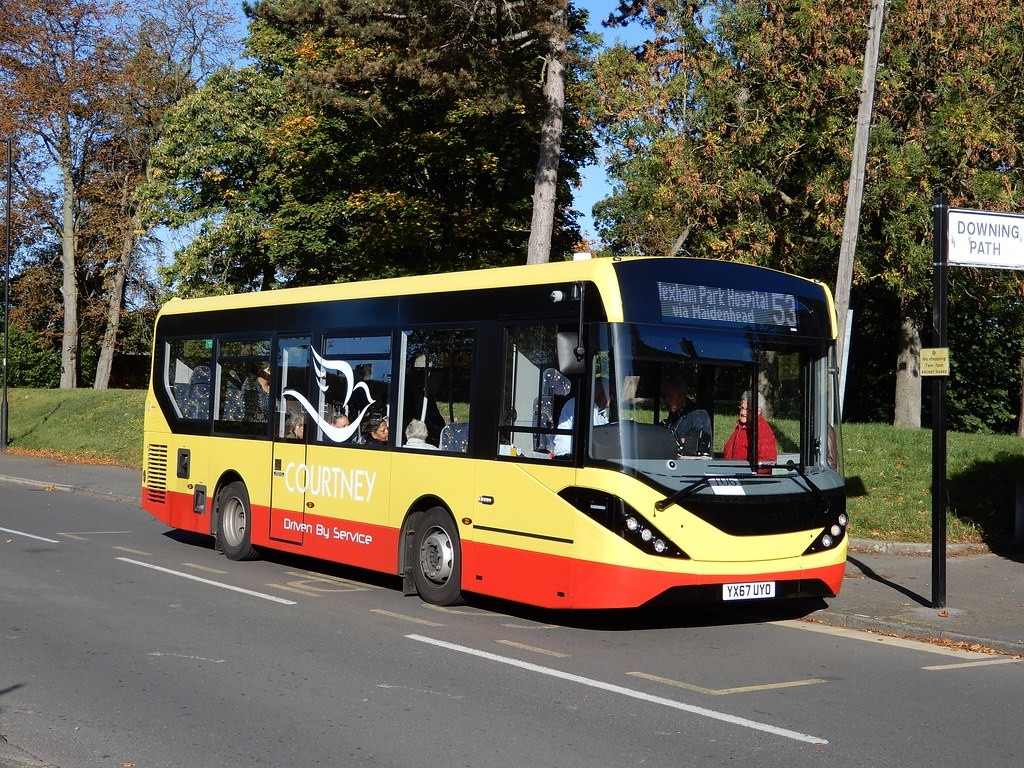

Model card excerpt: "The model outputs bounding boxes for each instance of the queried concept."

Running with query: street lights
[0,137,12,452]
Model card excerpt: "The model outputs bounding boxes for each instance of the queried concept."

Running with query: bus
[139,252,850,613]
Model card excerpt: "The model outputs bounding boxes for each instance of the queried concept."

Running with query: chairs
[532,368,571,455]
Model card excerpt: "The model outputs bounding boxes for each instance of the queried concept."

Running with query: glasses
[739,406,748,410]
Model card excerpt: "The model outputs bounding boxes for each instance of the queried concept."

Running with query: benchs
[173,366,250,422]
[440,422,469,453]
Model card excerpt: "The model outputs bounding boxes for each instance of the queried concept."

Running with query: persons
[361,418,388,444]
[553,377,610,456]
[826,423,837,473]
[286,417,304,439]
[241,362,270,423]
[663,384,714,457]
[401,419,439,450]
[323,414,349,443]
[723,392,777,474]
